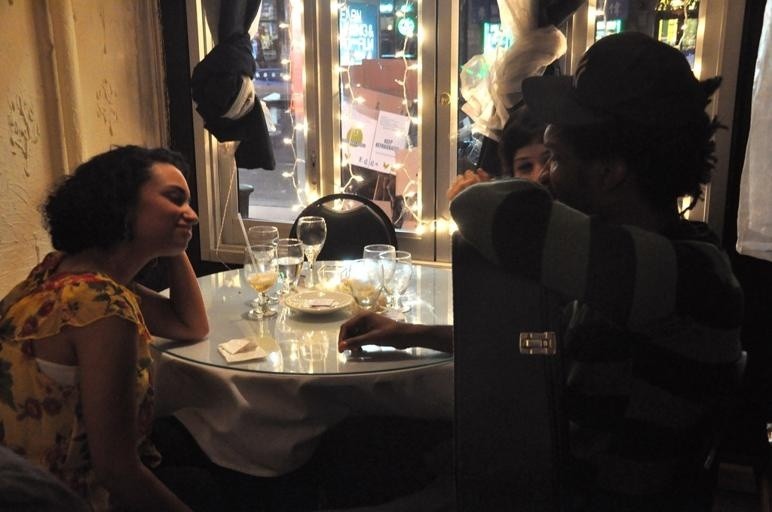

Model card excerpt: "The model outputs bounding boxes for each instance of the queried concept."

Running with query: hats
[520,29,713,134]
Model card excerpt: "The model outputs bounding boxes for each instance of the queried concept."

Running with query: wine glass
[243,215,413,319]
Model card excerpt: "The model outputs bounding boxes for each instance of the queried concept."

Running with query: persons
[338,30,746,512]
[0,143,209,510]
[448,104,549,201]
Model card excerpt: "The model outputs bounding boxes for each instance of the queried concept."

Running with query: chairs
[288,193,397,260]
[452,233,582,512]
